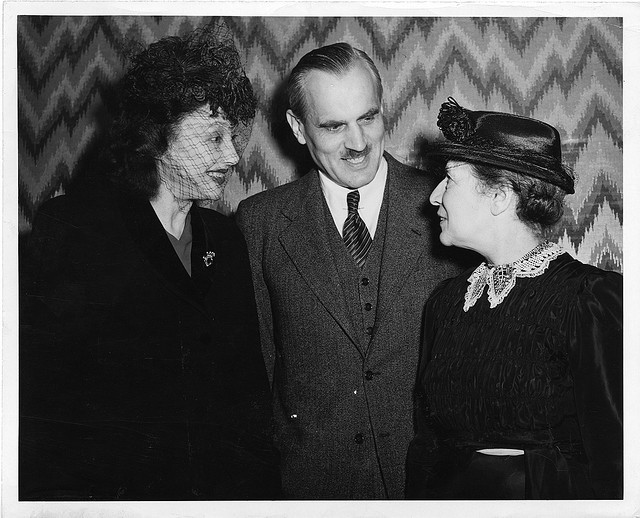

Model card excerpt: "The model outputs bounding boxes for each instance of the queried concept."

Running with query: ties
[344,191,374,268]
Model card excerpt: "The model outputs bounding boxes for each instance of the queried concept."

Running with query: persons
[232,41,477,499]
[404,95,623,499]
[20,35,281,500]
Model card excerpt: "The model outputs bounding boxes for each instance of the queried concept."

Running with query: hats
[435,95,578,196]
